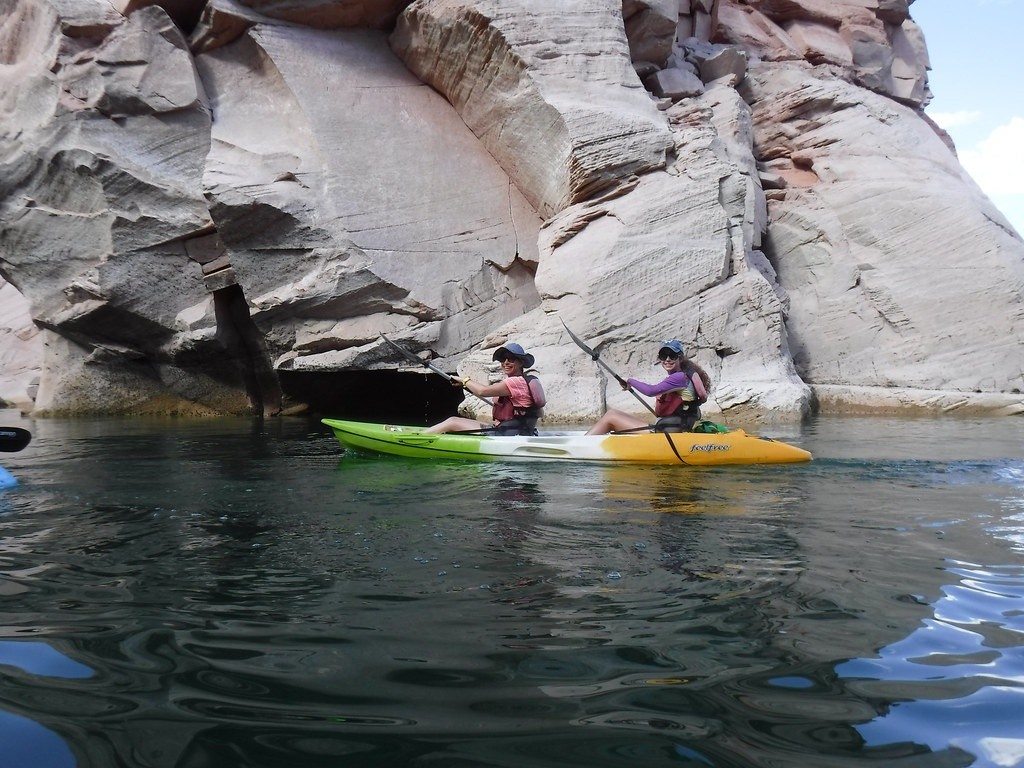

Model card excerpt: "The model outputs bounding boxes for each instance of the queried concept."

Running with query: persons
[584,340,710,435]
[383,343,547,436]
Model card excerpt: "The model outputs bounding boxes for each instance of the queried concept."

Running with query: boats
[0,466,19,492]
[320,417,815,467]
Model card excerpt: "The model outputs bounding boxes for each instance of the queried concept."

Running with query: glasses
[658,352,682,361]
[498,353,518,363]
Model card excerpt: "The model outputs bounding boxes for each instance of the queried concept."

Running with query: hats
[658,339,684,355]
[492,343,534,368]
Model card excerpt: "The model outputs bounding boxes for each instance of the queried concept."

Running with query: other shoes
[383,424,403,432]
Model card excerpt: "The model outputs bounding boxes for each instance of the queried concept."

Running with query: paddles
[558,315,657,419]
[378,329,494,407]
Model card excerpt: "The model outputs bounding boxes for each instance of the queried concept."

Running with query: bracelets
[462,376,470,388]
